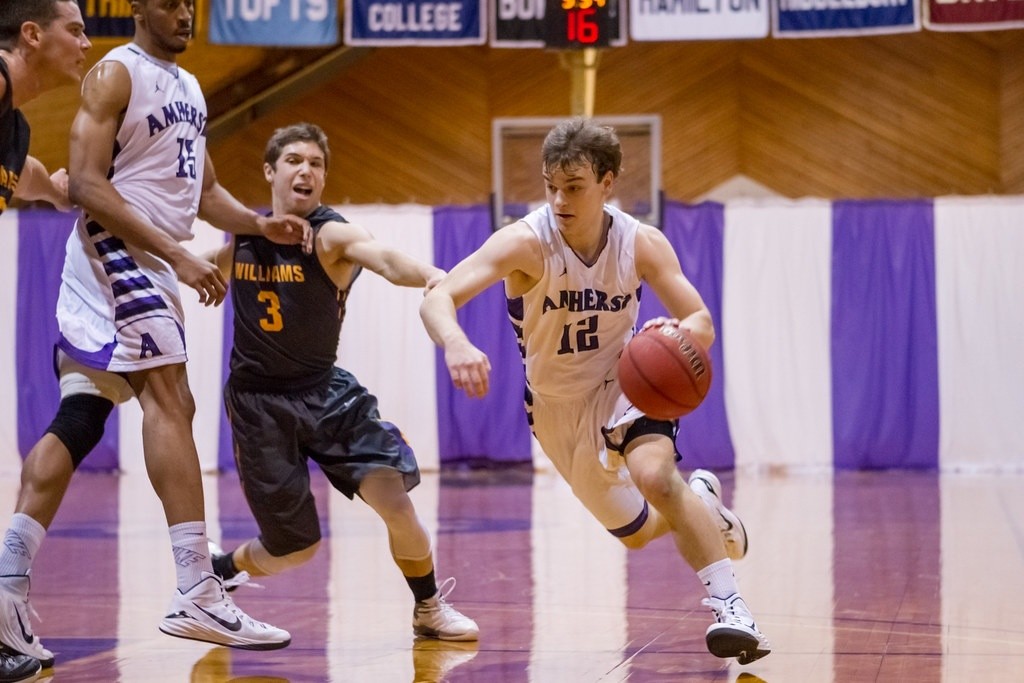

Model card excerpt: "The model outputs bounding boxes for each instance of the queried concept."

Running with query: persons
[0,0,314,683]
[178,122,481,641]
[420,116,771,665]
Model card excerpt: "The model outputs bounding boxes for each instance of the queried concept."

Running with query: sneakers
[159,571,291,651]
[705,593,771,665]
[412,591,479,640]
[207,538,239,592]
[0,574,55,667]
[688,469,748,561]
[413,638,478,680]
[0,652,42,683]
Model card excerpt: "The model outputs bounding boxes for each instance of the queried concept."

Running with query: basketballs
[618,322,714,420]
